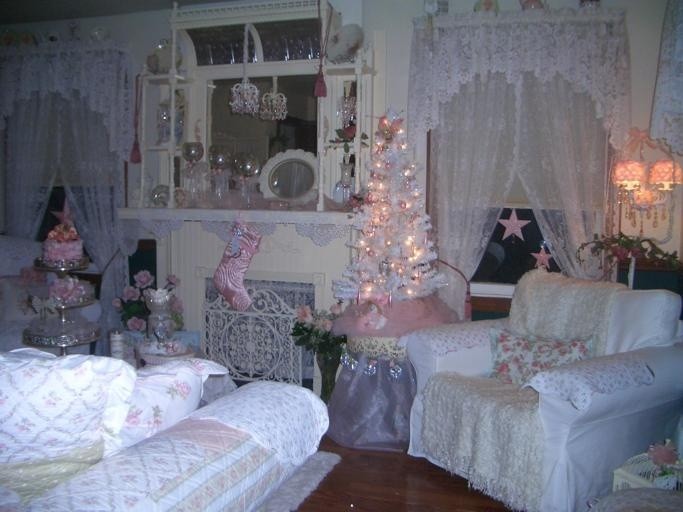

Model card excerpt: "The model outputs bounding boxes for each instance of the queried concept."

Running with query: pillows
[488,326,585,387]
[115,357,227,447]
[0,348,132,490]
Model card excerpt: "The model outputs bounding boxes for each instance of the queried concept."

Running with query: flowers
[329,126,355,164]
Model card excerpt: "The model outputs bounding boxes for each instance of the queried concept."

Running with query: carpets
[255,452,342,512]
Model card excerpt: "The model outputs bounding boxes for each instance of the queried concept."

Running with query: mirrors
[258,147,318,207]
[204,72,318,207]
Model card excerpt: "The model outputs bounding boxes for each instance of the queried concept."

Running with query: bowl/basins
[138,341,195,365]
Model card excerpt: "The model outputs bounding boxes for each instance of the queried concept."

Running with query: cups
[198,33,318,64]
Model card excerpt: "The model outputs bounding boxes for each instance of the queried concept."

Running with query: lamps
[612,129,683,286]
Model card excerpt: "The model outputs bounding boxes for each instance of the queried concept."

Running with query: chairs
[407,270,683,512]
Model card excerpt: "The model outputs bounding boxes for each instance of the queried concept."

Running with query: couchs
[0,339,329,512]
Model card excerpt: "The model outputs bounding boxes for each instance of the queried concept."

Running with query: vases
[332,162,354,204]
